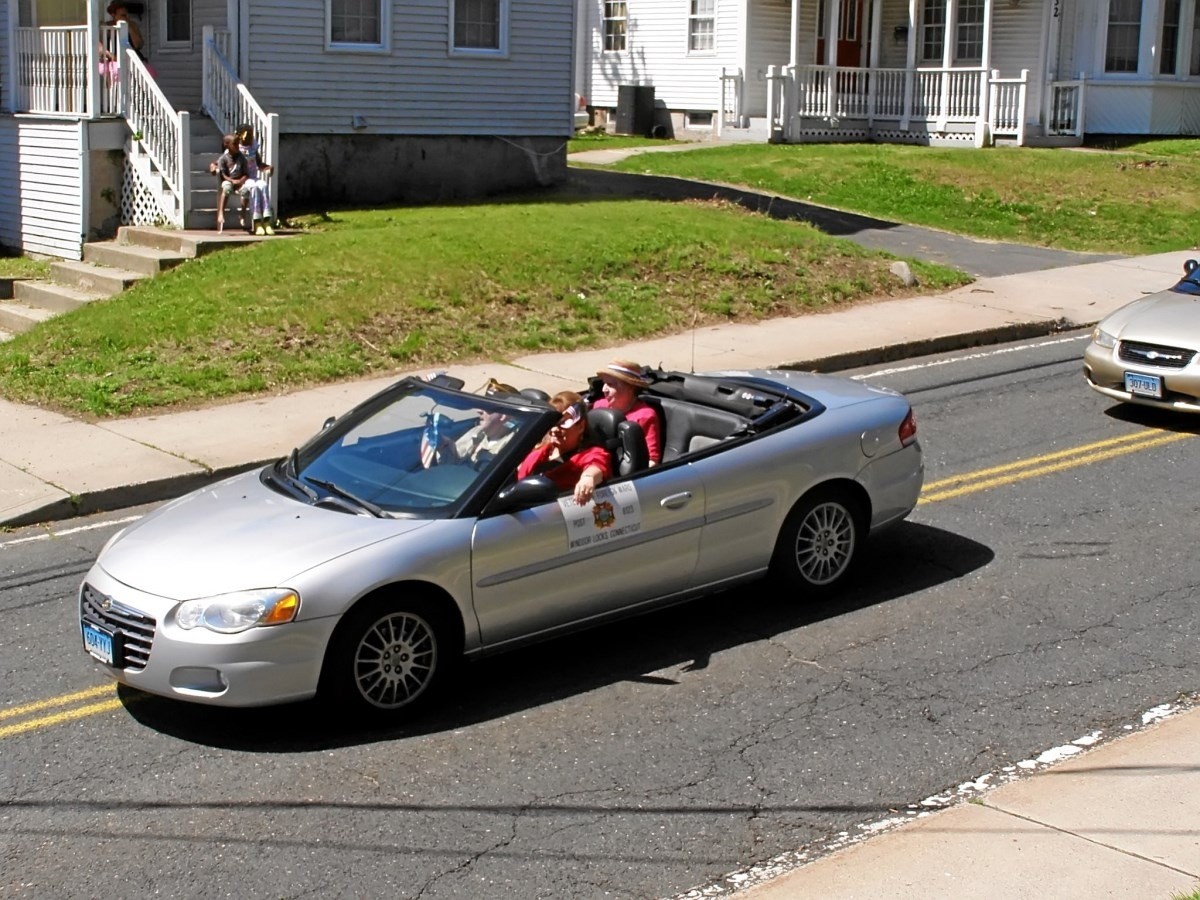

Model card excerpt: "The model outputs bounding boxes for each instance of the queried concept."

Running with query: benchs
[634,395,745,464]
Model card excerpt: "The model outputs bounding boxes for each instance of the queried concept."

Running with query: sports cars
[71,368,924,742]
[1079,256,1200,420]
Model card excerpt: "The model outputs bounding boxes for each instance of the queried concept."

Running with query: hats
[596,359,654,388]
[486,382,522,396]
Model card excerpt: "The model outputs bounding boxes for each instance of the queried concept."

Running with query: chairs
[581,409,645,477]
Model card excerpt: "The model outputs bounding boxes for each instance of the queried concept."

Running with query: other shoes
[218,213,224,223]
[263,222,274,235]
[239,214,246,226]
[255,222,265,235]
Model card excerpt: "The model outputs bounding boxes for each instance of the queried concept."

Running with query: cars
[575,92,590,133]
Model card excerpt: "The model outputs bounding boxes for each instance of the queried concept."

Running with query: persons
[216,134,251,223]
[429,382,524,464]
[99,0,144,113]
[517,390,610,507]
[210,125,275,235]
[590,360,662,471]
